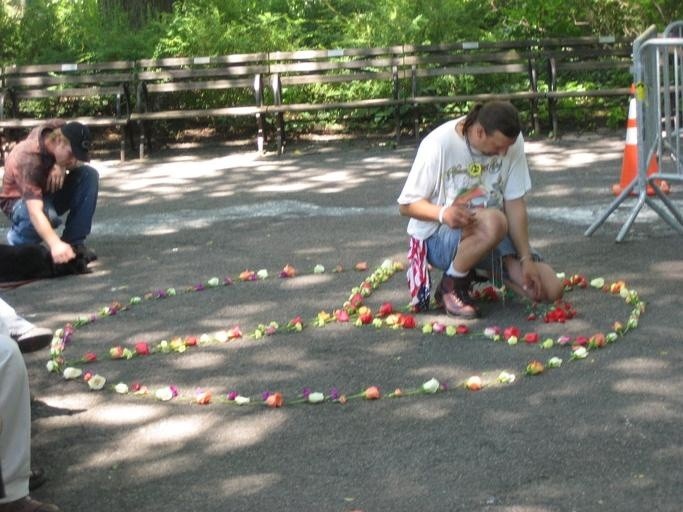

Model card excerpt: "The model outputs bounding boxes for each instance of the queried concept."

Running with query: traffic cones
[612,84,670,196]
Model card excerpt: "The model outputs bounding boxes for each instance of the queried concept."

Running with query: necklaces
[466,140,483,176]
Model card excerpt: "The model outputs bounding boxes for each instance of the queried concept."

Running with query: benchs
[0,34,679,161]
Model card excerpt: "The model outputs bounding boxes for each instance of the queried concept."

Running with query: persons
[0,297,52,354]
[0,329,63,510]
[0,118,98,267]
[396,100,563,322]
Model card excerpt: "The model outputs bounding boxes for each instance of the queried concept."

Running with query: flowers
[46,261,418,407]
[390,270,644,401]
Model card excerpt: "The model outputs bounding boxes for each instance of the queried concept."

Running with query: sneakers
[16,326,52,354]
[0,465,59,511]
[58,238,97,261]
[435,270,488,319]
[6,229,16,247]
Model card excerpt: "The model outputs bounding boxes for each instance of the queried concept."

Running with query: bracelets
[516,254,536,266]
[438,206,449,224]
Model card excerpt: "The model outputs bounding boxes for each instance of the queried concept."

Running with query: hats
[60,121,92,163]
